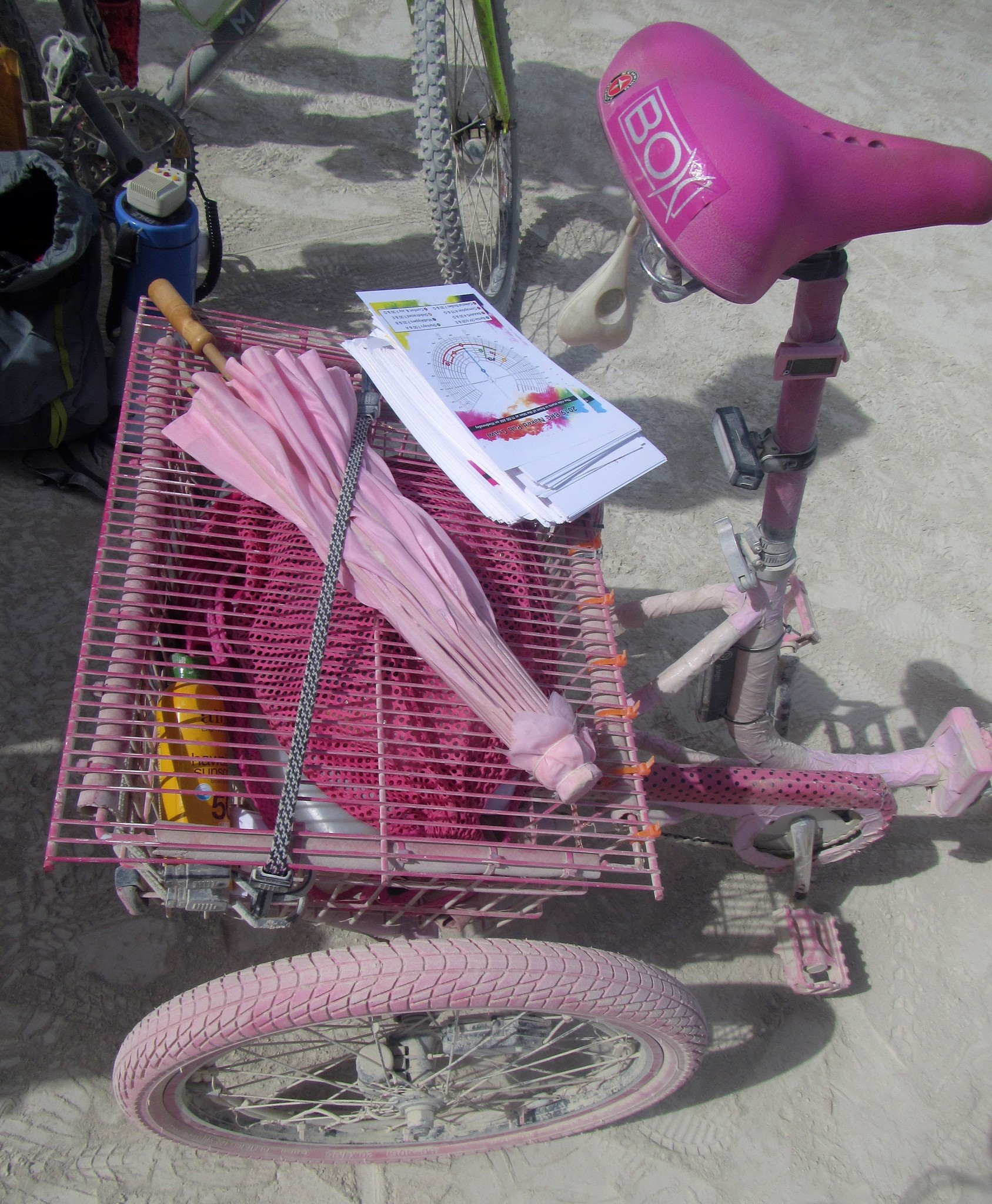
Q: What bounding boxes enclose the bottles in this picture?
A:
[157,652,232,828]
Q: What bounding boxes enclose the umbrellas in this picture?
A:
[148,278,602,807]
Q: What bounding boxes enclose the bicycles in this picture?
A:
[0,1,527,330]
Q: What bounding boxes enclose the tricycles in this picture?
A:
[32,17,991,1166]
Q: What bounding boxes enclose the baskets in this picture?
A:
[94,327,644,935]
